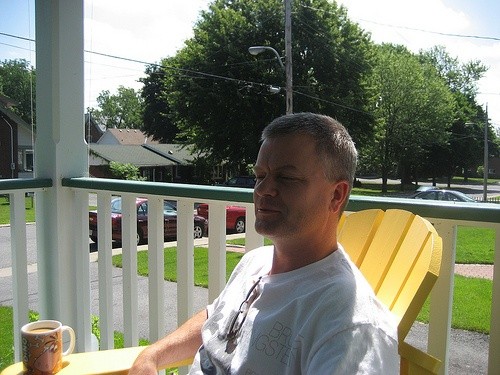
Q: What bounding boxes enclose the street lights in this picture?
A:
[248,45,293,115]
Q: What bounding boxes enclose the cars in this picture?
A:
[197,203,245,232]
[89,198,207,245]
[408,190,479,203]
[415,186,440,193]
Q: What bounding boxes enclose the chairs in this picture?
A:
[0,208,445,375]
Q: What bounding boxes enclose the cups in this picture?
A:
[21,319,76,375]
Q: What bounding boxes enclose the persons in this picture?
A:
[127,111,399,375]
